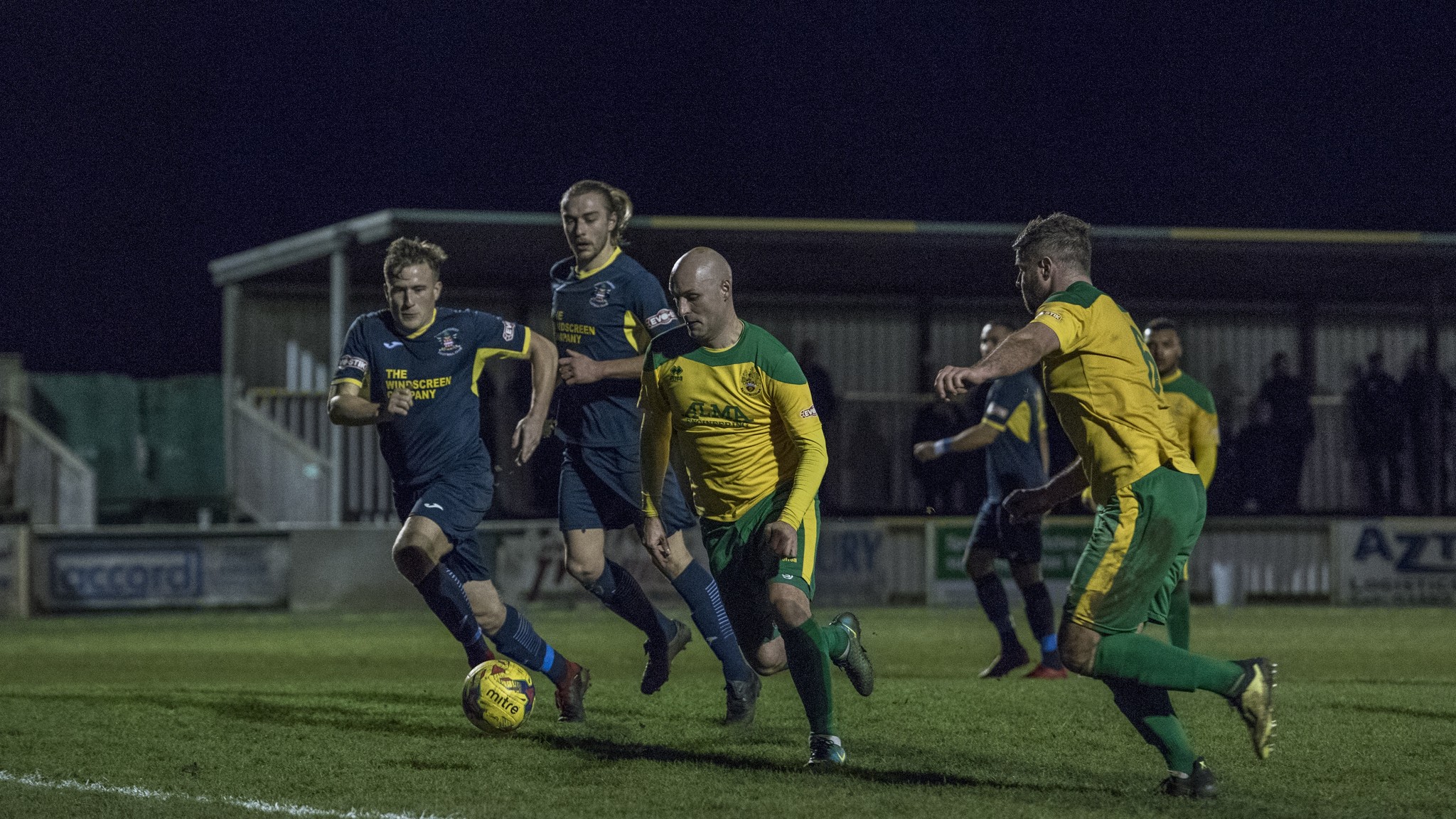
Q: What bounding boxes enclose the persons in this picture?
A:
[637,244,877,766]
[791,337,840,518]
[1136,316,1221,652]
[929,206,1274,797]
[318,232,595,722]
[908,387,977,512]
[1221,337,1455,514]
[907,319,1069,680]
[537,174,765,730]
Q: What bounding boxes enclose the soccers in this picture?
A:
[461,659,536,739]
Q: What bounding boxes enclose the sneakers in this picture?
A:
[831,614,870,697]
[977,647,1030,678]
[638,621,692,693]
[722,665,765,730]
[1163,755,1219,797]
[1025,662,1069,679]
[558,662,592,722]
[804,740,849,770]
[1229,654,1277,759]
